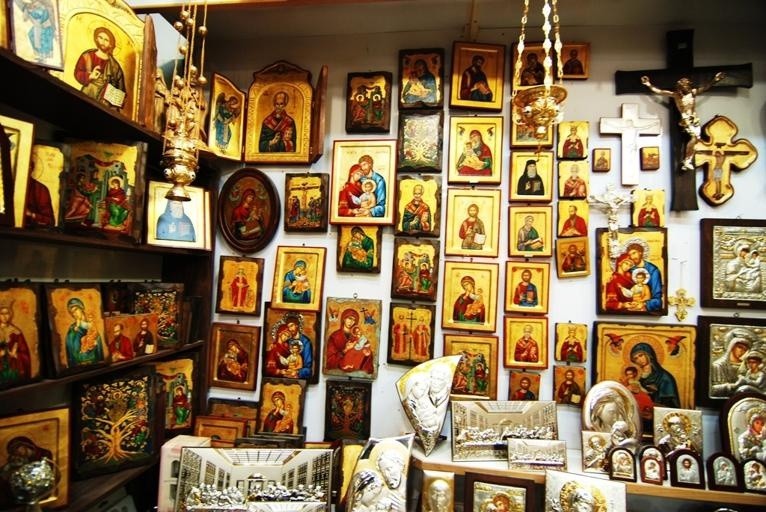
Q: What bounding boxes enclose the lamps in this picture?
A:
[136,13,188,68]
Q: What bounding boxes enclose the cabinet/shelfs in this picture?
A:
[0,46,214,512]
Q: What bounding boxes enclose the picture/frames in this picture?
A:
[127,314,158,357]
[216,168,281,254]
[0,115,33,227]
[130,282,186,350]
[391,236,441,301]
[607,448,637,483]
[450,401,558,462]
[58,137,147,243]
[208,323,262,391]
[640,146,659,170]
[463,471,535,511]
[669,452,704,488]
[631,188,667,226]
[557,121,589,161]
[193,415,248,447]
[21,144,64,228]
[105,316,134,364]
[508,206,553,259]
[553,366,587,408]
[174,446,333,512]
[206,398,261,438]
[6,0,63,72]
[420,469,455,512]
[545,470,626,512]
[591,148,610,172]
[508,370,541,401]
[336,225,383,274]
[700,218,766,311]
[557,41,590,80]
[448,115,502,185]
[397,46,444,113]
[396,355,459,456]
[323,297,382,379]
[329,140,397,222]
[395,175,442,240]
[44,284,111,379]
[1,281,45,390]
[718,392,766,466]
[556,201,589,238]
[341,434,415,512]
[234,438,285,448]
[509,150,554,204]
[345,70,393,134]
[144,180,205,251]
[508,439,567,472]
[582,381,641,457]
[741,459,766,492]
[443,334,497,403]
[554,323,587,364]
[503,315,549,371]
[65,369,159,482]
[249,435,301,449]
[50,0,157,133]
[0,405,72,512]
[149,358,197,438]
[216,256,265,317]
[638,445,668,479]
[259,432,305,448]
[395,111,444,175]
[592,321,699,436]
[323,379,372,446]
[641,457,661,483]
[707,452,744,493]
[443,260,499,334]
[512,42,554,92]
[510,97,554,150]
[696,315,766,410]
[386,302,437,366]
[244,61,327,166]
[450,41,504,111]
[653,408,702,470]
[208,73,245,163]
[555,237,590,278]
[582,431,612,476]
[595,227,667,317]
[505,260,550,315]
[255,377,307,435]
[445,187,501,257]
[558,160,591,200]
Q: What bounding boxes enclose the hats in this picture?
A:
[631,267,650,284]
[627,244,645,252]
[271,392,286,403]
[460,275,475,285]
[289,340,304,355]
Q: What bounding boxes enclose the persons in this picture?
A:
[342,227,377,271]
[402,186,431,232]
[6,433,53,464]
[519,216,543,251]
[156,200,194,240]
[489,495,510,512]
[513,325,539,362]
[511,377,534,400]
[76,25,128,107]
[347,80,383,124]
[618,268,651,312]
[721,235,763,290]
[606,255,635,312]
[640,71,725,171]
[402,59,438,104]
[521,52,545,87]
[287,182,325,230]
[227,267,253,310]
[282,254,312,303]
[713,329,766,398]
[709,150,726,200]
[563,244,584,272]
[396,249,432,296]
[0,296,31,381]
[217,338,251,382]
[646,462,657,478]
[626,243,664,315]
[656,415,699,453]
[23,0,53,62]
[350,179,377,218]
[461,54,494,103]
[338,165,361,217]
[564,327,583,360]
[107,323,134,361]
[564,126,584,156]
[63,297,104,368]
[452,276,488,323]
[738,410,766,458]
[457,126,495,180]
[394,313,429,357]
[562,205,586,236]
[749,464,765,488]
[104,178,130,226]
[458,204,486,250]
[515,270,538,307]
[518,160,545,195]
[172,384,191,428]
[559,370,579,400]
[583,435,610,464]
[71,308,100,353]
[24,165,53,229]
[261,90,302,155]
[564,165,586,197]
[638,196,659,226]
[597,150,608,168]
[260,388,293,432]
[619,366,656,415]
[453,350,489,393]
[645,152,658,168]
[717,461,730,484]
[230,182,265,240]
[358,155,387,217]
[134,319,155,358]
[265,311,314,376]
[630,342,680,410]
[213,89,238,155]
[561,47,581,76]
[67,174,90,225]
[678,459,696,481]
[327,305,376,375]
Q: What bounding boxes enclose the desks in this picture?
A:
[409,441,766,512]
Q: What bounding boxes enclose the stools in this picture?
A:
[158,435,211,512]
